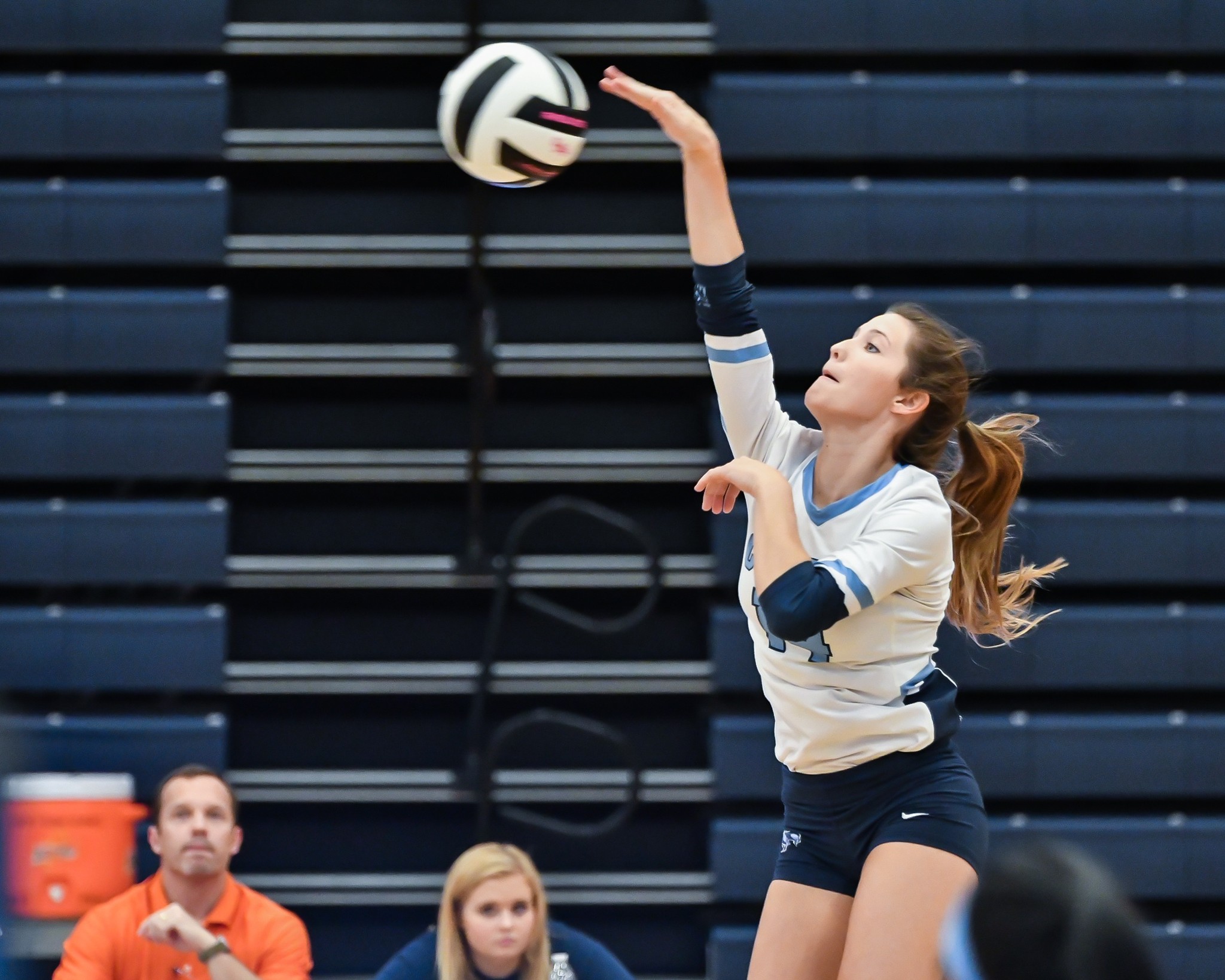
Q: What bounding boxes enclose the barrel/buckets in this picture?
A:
[0,773,146,917]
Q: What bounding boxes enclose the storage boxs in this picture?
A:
[1,772,149,919]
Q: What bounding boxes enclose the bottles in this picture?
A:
[548,952,577,980]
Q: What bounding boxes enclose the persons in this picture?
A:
[600,63,1071,980]
[51,766,313,980]
[366,842,639,980]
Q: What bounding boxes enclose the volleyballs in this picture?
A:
[437,42,590,188]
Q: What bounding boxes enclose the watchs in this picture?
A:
[197,936,231,964]
